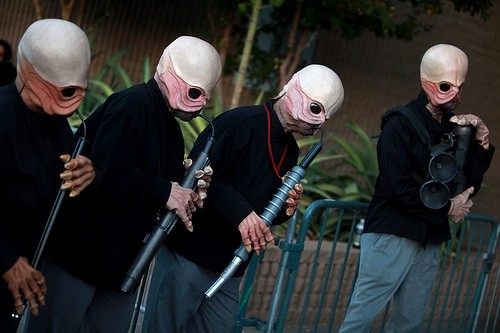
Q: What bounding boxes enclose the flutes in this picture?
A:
[119,115,216,293]
[205,127,324,299]
[5,109,86,333]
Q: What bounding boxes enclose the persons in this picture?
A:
[129,64,345,333]
[24,35,223,333]
[0,39,17,86]
[337,44,496,333]
[0,18,96,333]
